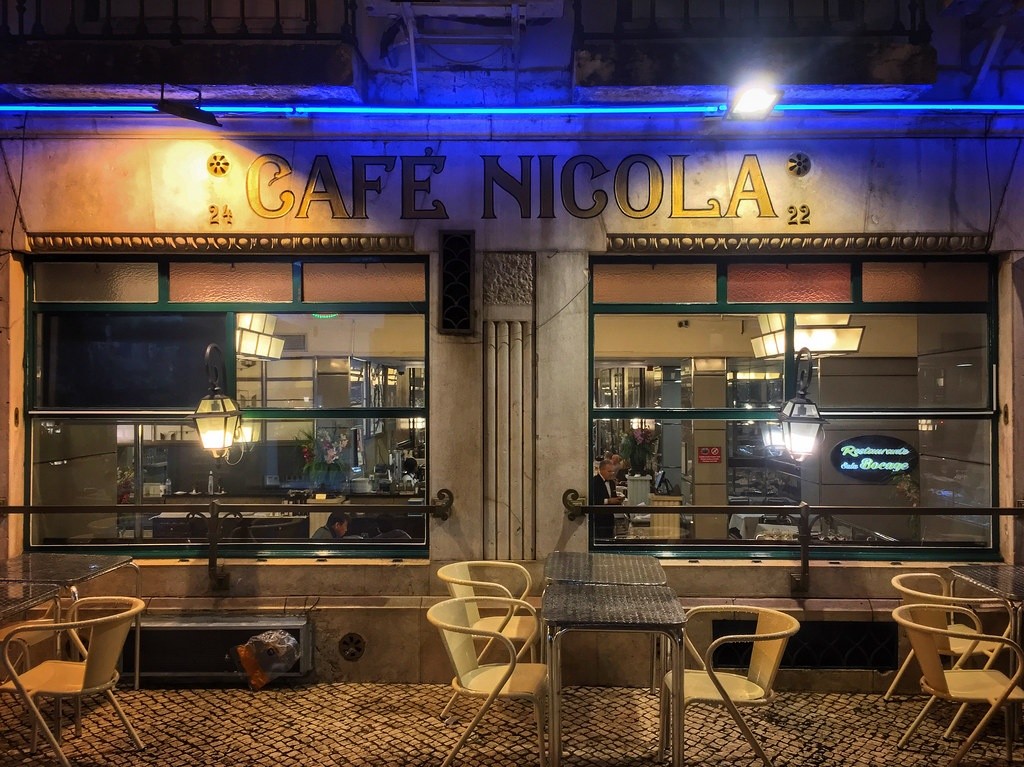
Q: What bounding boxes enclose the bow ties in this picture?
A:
[605,480,610,482]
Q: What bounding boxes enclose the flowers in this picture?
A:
[620,428,658,460]
[294,422,355,481]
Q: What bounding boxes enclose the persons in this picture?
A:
[612,454,628,481]
[310,511,349,539]
[593,460,624,538]
[401,458,420,488]
[604,451,614,460]
[374,514,412,538]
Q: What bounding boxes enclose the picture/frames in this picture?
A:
[316,426,358,467]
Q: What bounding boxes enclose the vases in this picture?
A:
[312,476,337,498]
[628,456,646,477]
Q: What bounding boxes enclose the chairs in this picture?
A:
[891,603,1024,767]
[762,497,791,507]
[437,561,547,725]
[727,496,749,506]
[657,604,801,767]
[758,515,793,526]
[0,596,146,767]
[426,596,550,767]
[883,573,1012,740]
[0,619,66,726]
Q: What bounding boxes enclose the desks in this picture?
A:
[0,580,63,747]
[540,584,688,767]
[152,512,310,539]
[948,565,1024,741]
[624,493,991,540]
[0,552,141,738]
[328,495,427,525]
[542,551,671,749]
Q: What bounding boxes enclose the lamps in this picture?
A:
[235,313,285,361]
[191,342,244,461]
[237,392,263,454]
[152,77,223,128]
[722,71,785,121]
[350,367,398,387]
[750,314,865,361]
[779,347,831,462]
[759,363,786,456]
